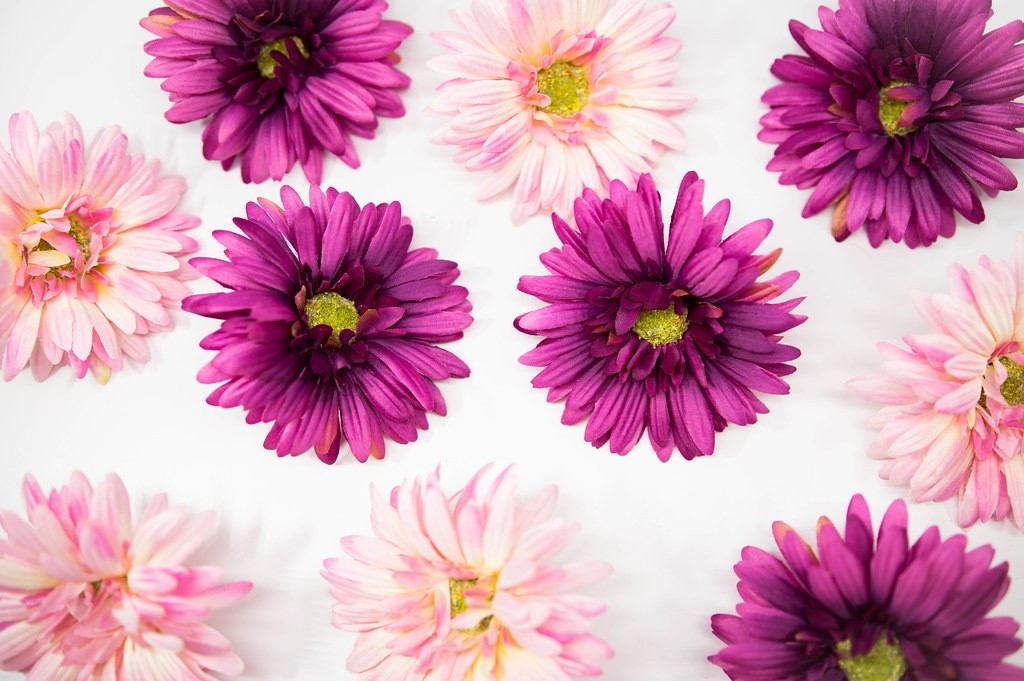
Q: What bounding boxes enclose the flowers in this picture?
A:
[427,1,696,228]
[317,457,617,681]
[0,467,258,681]
[179,183,477,468]
[0,109,206,392]
[753,1,1023,253]
[839,236,1024,537]
[704,492,1024,681]
[138,0,415,185]
[511,172,808,465]
